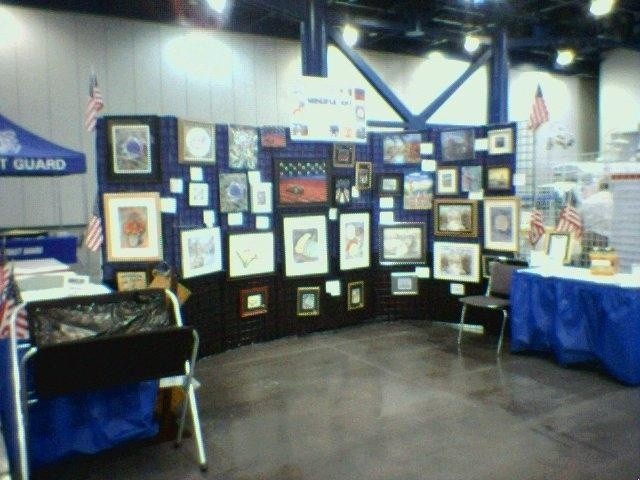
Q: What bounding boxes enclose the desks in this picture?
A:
[14,285,207,480]
[507,264,640,385]
[0,231,174,471]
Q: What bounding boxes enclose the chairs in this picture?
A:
[458,258,529,356]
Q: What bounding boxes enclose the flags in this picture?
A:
[558,190,583,237]
[83,70,105,131]
[527,203,544,244]
[0,238,32,339]
[527,84,550,130]
[83,197,105,252]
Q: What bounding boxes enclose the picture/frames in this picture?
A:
[543,228,574,262]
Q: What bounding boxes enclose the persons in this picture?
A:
[580,177,611,248]
